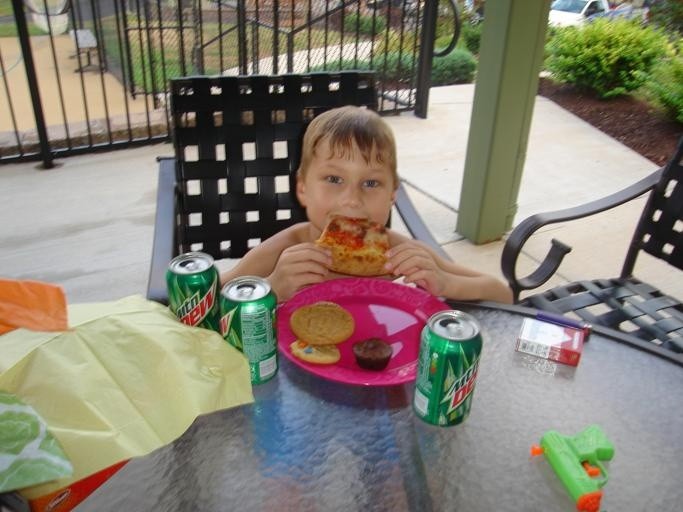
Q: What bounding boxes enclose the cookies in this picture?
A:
[290,342,340,365]
[289,302,354,344]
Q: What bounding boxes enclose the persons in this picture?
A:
[215,106,516,306]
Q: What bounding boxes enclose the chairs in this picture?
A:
[145,72,457,306]
[501,136,683,354]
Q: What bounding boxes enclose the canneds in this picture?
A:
[413,308,482,426]
[165,253,220,334]
[220,275,279,387]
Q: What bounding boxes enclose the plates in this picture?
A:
[277,278,454,385]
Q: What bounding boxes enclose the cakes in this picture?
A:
[351,338,393,370]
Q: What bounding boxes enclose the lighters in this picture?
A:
[535,310,592,338]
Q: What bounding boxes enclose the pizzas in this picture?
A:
[315,216,390,277]
